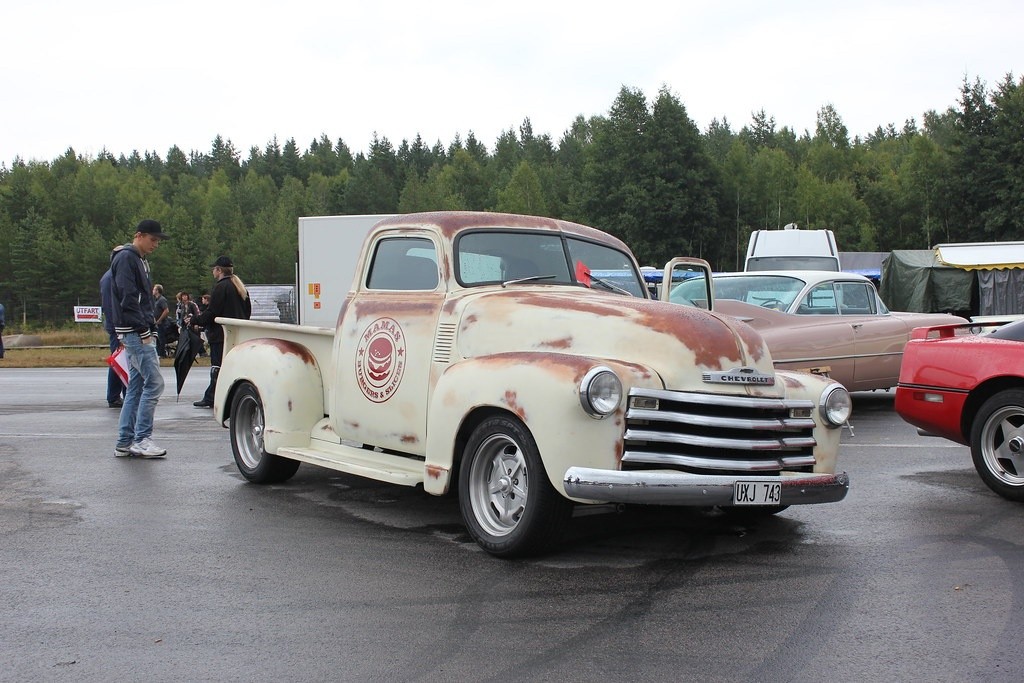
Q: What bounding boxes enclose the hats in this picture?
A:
[110,245,125,262]
[137,220,170,241]
[208,255,233,267]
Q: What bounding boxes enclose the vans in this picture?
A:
[745,225,842,318]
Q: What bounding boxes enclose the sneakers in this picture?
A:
[115,446,139,457]
[129,436,167,456]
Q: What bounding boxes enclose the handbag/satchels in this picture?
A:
[163,315,179,344]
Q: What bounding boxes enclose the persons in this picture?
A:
[99,220,172,457]
[184,256,251,408]
[152,284,211,359]
[0,303,5,359]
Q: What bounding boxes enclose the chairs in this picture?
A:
[505,259,540,281]
[392,256,437,290]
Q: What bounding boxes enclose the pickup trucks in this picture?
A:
[215,209,851,557]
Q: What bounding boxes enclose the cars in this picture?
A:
[893,313,1023,503]
[575,265,717,304]
[657,270,973,402]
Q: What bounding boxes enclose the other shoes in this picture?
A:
[193,398,214,408]
[158,354,170,359]
[108,397,124,408]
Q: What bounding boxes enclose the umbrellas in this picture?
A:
[107,344,129,389]
[174,316,204,402]
